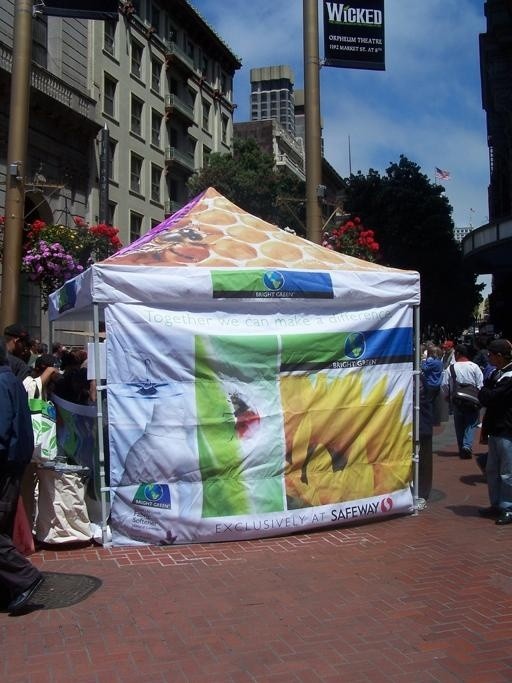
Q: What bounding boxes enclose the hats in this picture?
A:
[442,340,453,348]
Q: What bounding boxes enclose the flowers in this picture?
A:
[20,213,125,311]
[323,211,382,265]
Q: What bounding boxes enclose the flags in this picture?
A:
[435,166,451,179]
[322,0,387,71]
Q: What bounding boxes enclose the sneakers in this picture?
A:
[8,572,45,613]
[418,498,427,510]
[496,513,511,526]
[479,507,496,516]
[462,448,472,459]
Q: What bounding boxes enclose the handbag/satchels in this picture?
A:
[450,364,482,420]
[29,378,57,463]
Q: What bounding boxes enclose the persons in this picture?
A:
[474,337,511,528]
[0,335,47,614]
[0,314,111,487]
[413,319,498,497]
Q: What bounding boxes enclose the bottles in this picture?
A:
[110,403,203,543]
